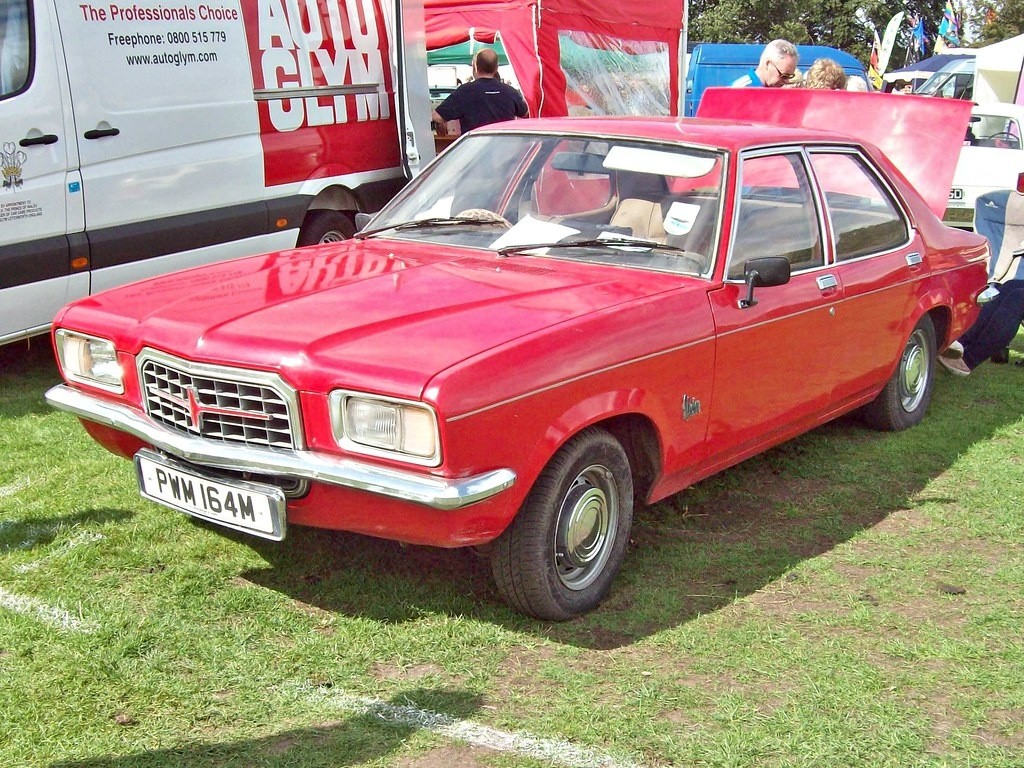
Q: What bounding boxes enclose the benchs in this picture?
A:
[613,195,810,269]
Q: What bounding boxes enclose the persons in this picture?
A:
[432,48,530,138]
[934,279,1024,376]
[726,39,856,206]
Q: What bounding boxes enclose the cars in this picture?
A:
[944,103,1024,230]
[883,48,975,102]
[46,83,1001,624]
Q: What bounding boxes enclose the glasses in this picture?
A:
[771,61,795,79]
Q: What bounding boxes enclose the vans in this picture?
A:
[683,43,877,120]
[0,0,418,348]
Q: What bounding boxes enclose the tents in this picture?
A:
[972,32,1024,139]
[401,0,691,158]
[881,46,979,102]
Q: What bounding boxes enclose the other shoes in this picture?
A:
[941,340,964,358]
[936,354,971,377]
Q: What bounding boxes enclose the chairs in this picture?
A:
[974,189,1024,368]
